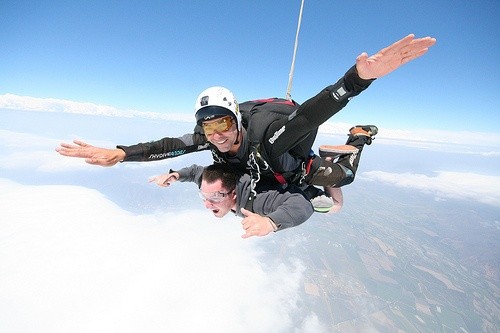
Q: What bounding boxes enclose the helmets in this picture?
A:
[194,87,242,131]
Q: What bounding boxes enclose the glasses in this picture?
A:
[202,117,234,135]
[199,188,235,202]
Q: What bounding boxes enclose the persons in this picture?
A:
[55,34,439,188]
[147,145,360,239]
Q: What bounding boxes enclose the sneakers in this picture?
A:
[319,145,359,163]
[349,125,378,145]
